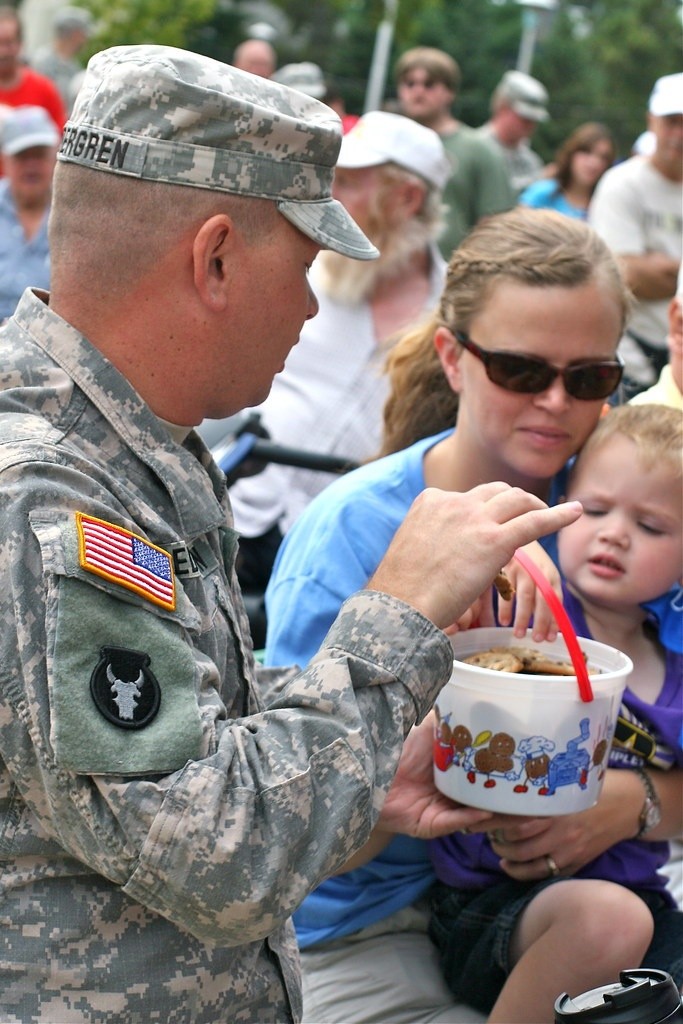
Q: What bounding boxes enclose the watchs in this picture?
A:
[632,767,663,840]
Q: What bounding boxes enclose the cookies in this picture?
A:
[456,645,604,676]
[493,567,515,602]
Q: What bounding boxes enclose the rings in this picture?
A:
[545,856,559,876]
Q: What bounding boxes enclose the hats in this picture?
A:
[650,74,683,117]
[57,44,381,260]
[0,106,58,155]
[633,132,657,156]
[334,111,453,190]
[497,70,551,123]
[268,61,326,98]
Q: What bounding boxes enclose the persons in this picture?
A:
[394,46,515,262]
[426,403,683,1024]
[232,38,276,78]
[585,72,683,406]
[0,5,66,136]
[0,103,61,322]
[30,6,95,95]
[515,122,616,219]
[228,111,453,595]
[479,70,557,190]
[262,207,683,1024]
[0,44,584,1024]
[628,258,683,412]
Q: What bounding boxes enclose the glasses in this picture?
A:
[456,333,624,401]
[403,77,445,90]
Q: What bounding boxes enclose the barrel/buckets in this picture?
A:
[432,548,633,817]
